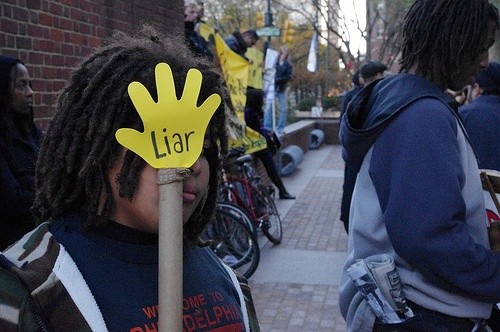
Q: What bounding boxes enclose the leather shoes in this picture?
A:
[279,191,295,199]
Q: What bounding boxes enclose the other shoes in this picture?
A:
[278,128,284,134]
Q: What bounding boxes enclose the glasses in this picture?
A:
[251,37,255,45]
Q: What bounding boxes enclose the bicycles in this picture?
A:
[193,147,283,279]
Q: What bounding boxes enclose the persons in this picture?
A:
[338,0,500,332]
[223,28,260,64]
[245,86,296,200]
[0,56,45,253]
[0,23,260,332]
[264,44,292,135]
